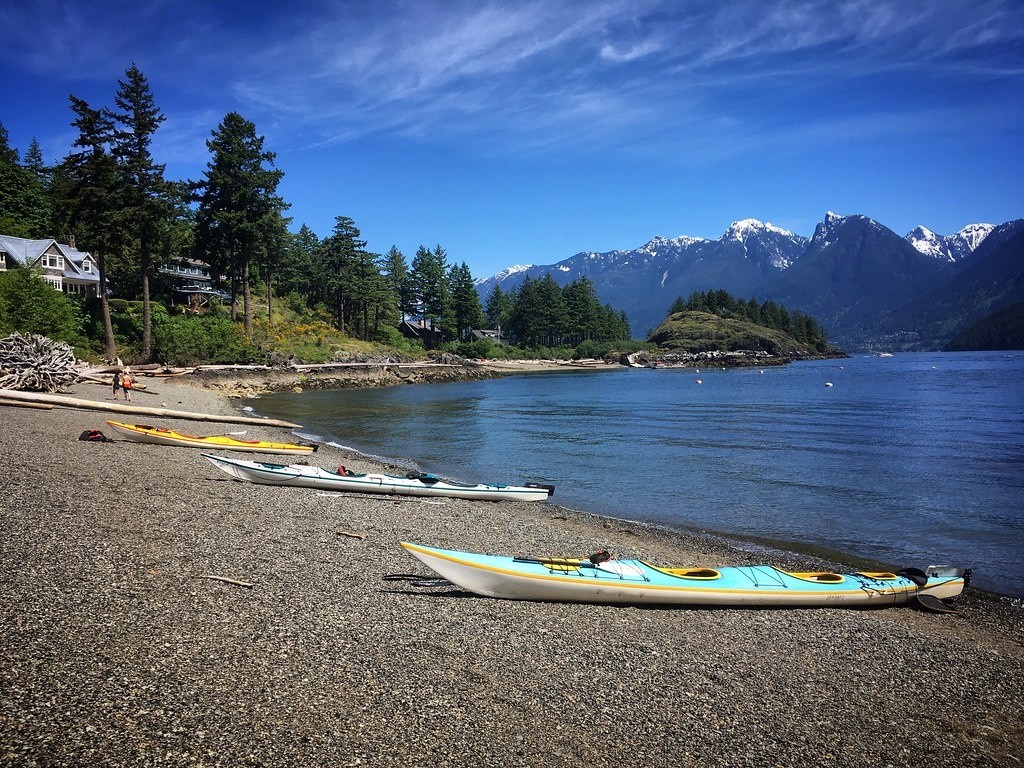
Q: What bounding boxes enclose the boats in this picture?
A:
[200,451,556,502]
[106,419,320,456]
[395,540,975,618]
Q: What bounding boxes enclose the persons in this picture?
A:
[113,374,119,400]
[123,371,131,401]
